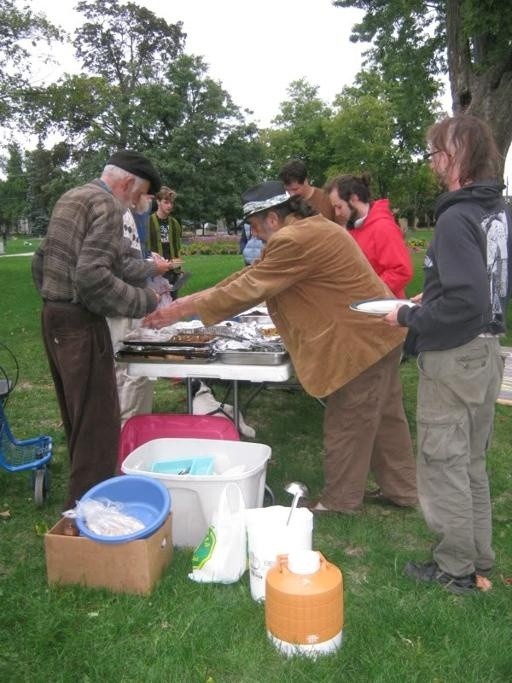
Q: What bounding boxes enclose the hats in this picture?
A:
[104,148,162,196]
[234,179,304,232]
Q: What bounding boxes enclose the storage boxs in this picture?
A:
[43,503,174,596]
[127,436,272,548]
[120,412,237,478]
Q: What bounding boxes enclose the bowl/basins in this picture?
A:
[73,476,171,542]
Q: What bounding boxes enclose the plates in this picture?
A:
[349,298,419,318]
[150,258,185,268]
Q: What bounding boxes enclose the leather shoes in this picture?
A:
[287,497,343,516]
[362,488,412,509]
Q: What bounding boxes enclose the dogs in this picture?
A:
[185,377,257,440]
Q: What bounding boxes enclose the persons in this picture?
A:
[325,174,413,298]
[31,149,160,517]
[368,115,512,589]
[141,180,419,513]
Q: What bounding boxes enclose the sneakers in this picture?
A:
[402,558,493,596]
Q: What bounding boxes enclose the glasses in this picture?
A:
[423,149,453,168]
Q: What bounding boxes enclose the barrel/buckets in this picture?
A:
[265,551,343,661]
[244,505,313,603]
